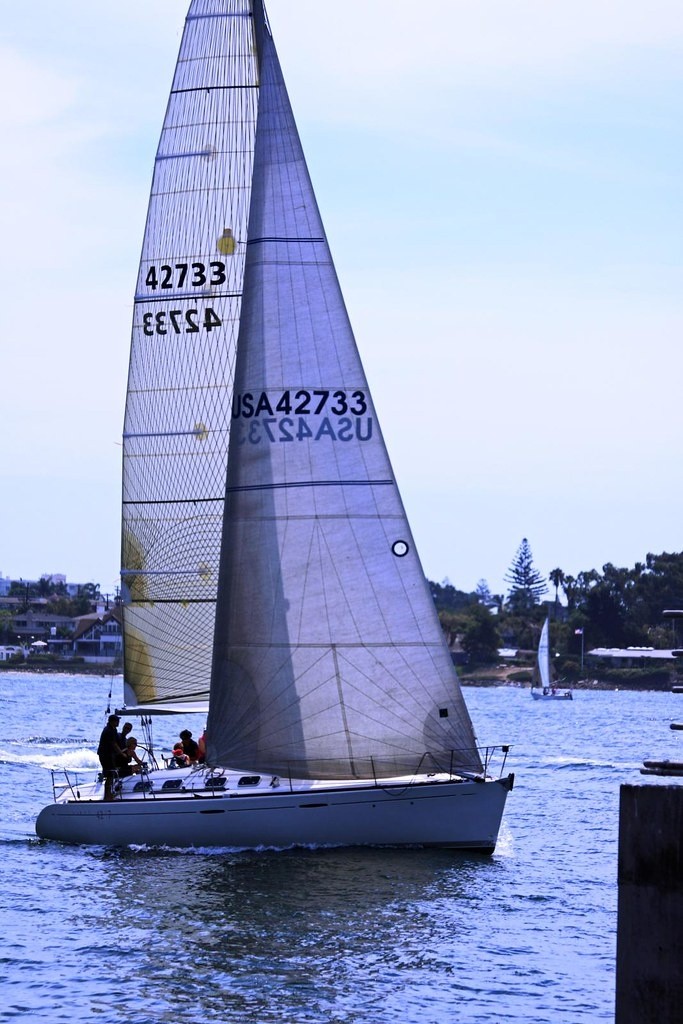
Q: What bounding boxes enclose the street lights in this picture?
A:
[17,634,35,649]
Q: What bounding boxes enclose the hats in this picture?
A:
[172,749,183,756]
[108,715,121,721]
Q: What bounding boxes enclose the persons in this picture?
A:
[552,684,556,695]
[97,714,149,800]
[543,686,549,695]
[173,724,206,768]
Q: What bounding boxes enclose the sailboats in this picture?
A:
[530,617,573,700]
[35,0,515,856]
